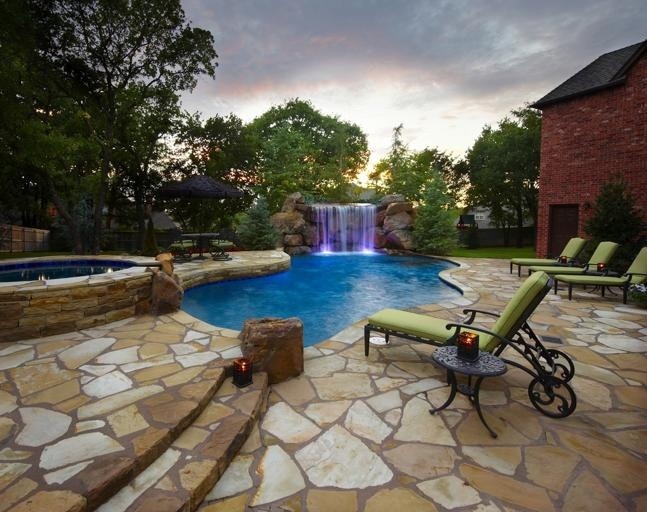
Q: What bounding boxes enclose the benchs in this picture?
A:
[459,215,479,231]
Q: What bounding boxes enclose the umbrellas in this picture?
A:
[157,174,244,255]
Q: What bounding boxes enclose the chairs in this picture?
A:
[206,239,234,261]
[363,271,576,419]
[170,226,191,268]
[510,238,647,305]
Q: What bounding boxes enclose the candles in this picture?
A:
[231,355,252,377]
[457,327,482,347]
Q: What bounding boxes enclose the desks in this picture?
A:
[180,230,219,260]
[430,346,508,442]
